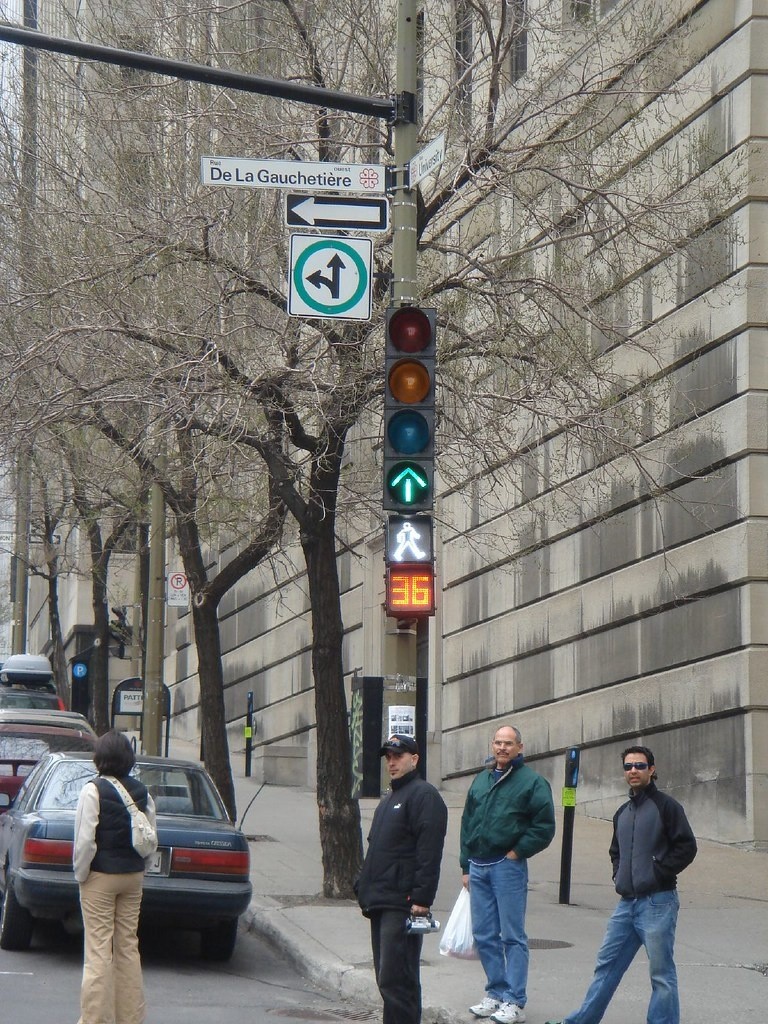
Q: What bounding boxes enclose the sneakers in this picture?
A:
[468,997,506,1016]
[489,1002,526,1024]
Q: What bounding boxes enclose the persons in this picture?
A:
[353,734,427,1024]
[458,725,555,1023]
[73,729,157,1024]
[558,746,698,1024]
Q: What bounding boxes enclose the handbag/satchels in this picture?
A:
[131,809,158,858]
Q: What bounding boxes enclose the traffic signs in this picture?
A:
[202,155,387,193]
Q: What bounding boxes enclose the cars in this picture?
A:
[0,654,66,714]
[0,750,255,971]
[1,707,107,816]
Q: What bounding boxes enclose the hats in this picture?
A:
[380,735,420,756]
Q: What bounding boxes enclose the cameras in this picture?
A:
[405,912,441,935]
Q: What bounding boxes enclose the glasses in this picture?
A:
[492,740,518,747]
[624,763,651,771]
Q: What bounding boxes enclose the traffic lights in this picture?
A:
[384,514,435,568]
[384,460,433,513]
[386,307,440,460]
[385,568,437,619]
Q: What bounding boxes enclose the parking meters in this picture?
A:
[557,745,585,904]
[243,690,253,776]
